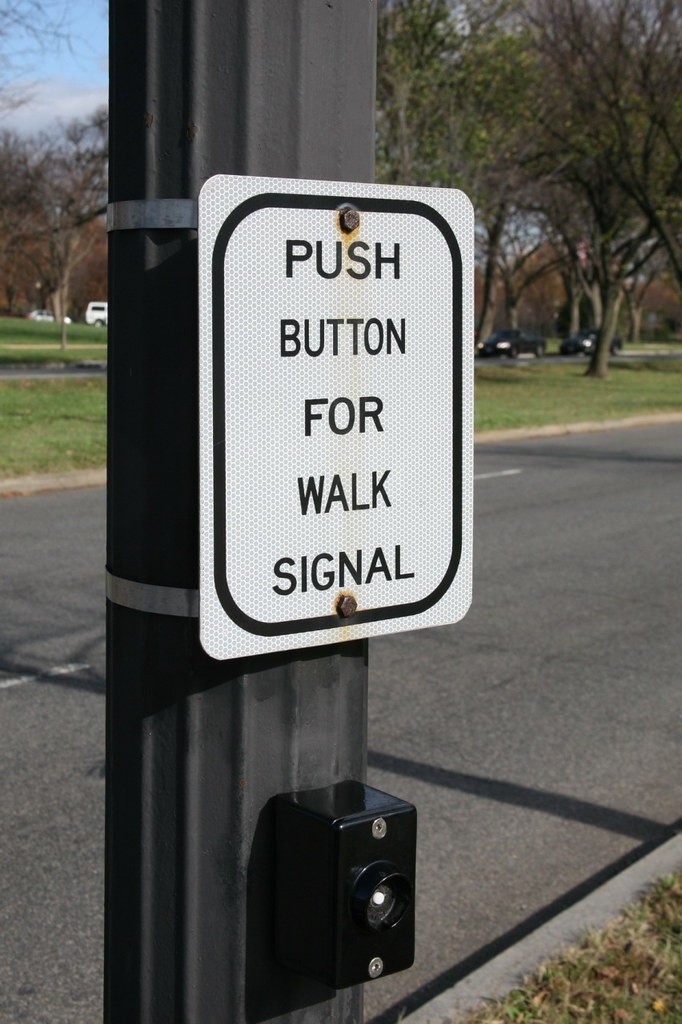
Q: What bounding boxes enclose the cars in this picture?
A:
[558,327,625,355]
[478,329,548,359]
[25,309,72,324]
[85,302,108,327]
